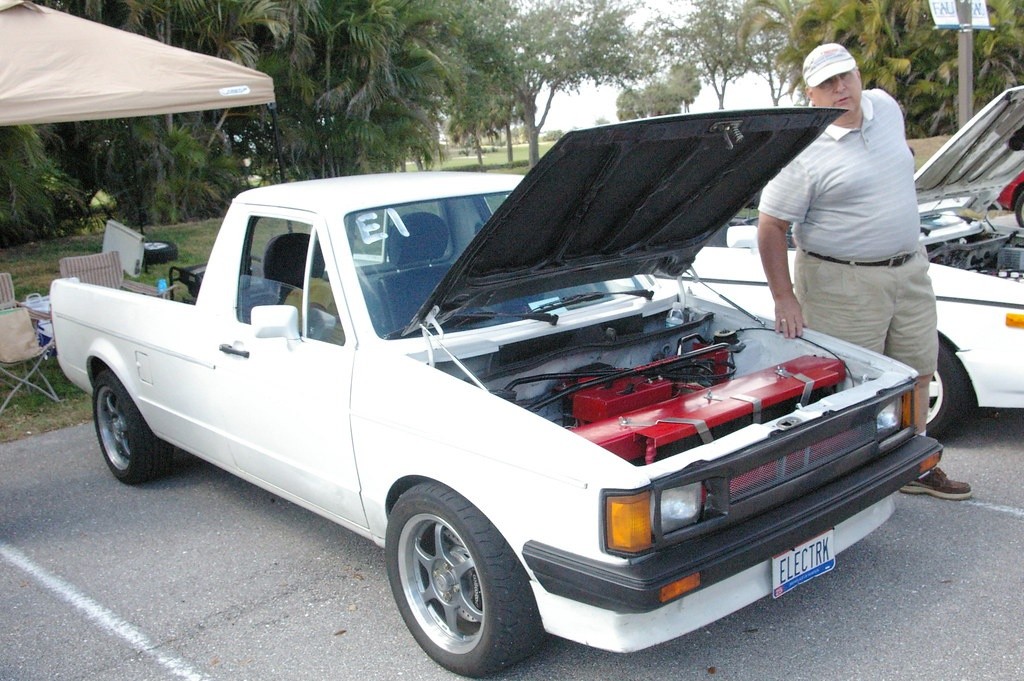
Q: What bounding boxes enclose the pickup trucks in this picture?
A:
[674,85,1024,446]
[47,105,943,681]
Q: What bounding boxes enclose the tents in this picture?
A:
[1,0,296,275]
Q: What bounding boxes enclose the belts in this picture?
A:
[802,249,917,266]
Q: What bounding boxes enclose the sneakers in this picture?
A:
[900,468,971,501]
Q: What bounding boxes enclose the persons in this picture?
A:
[757,42,973,500]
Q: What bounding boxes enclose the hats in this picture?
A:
[802,43,856,87]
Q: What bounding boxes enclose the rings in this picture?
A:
[781,319,786,322]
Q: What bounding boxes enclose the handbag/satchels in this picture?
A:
[0,296,43,363]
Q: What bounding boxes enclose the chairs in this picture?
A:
[247,232,345,347]
[0,272,60,414]
[59,250,171,301]
[378,213,453,336]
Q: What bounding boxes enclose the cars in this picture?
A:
[997,170,1024,229]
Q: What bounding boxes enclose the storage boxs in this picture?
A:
[21,292,57,356]
